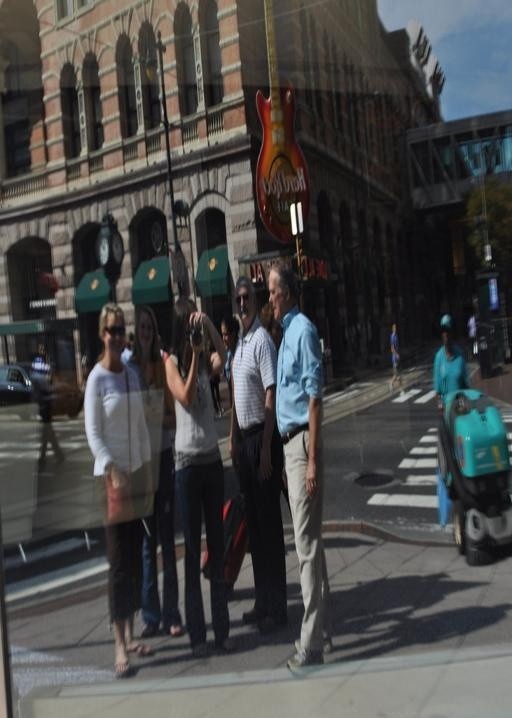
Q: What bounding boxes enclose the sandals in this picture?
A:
[114,642,153,677]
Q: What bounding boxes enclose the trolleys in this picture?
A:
[434,383,512,570]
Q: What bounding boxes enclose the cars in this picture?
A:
[0,360,84,423]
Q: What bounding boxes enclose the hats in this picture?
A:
[441,314,452,327]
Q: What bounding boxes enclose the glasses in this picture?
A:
[105,326,125,335]
[234,293,252,302]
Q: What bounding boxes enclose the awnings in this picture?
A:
[196,243,230,297]
[131,248,169,304]
[75,259,112,311]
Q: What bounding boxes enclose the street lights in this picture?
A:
[286,190,307,315]
[143,29,187,303]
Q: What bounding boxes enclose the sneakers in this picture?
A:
[285,631,332,677]
[242,610,289,632]
[140,623,182,637]
[190,635,237,655]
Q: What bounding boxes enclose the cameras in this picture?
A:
[190,327,202,346]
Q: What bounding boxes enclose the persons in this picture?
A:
[128,308,184,637]
[467,314,480,360]
[389,323,405,396]
[261,301,283,343]
[30,375,64,468]
[85,303,156,674]
[269,267,328,676]
[166,297,237,658]
[202,318,239,417]
[229,277,287,629]
[432,313,468,527]
